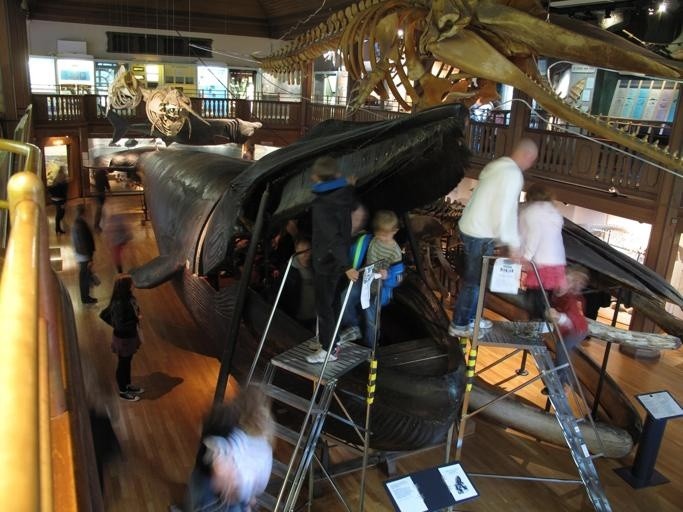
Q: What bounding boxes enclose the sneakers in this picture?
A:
[119,384,145,402]
[304,342,340,363]
[447,320,484,339]
[541,321,554,334]
[469,316,494,329]
[562,383,571,399]
[541,386,550,396]
[339,327,361,346]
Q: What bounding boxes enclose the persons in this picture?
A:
[299,154,360,363]
[49,167,67,234]
[89,172,110,230]
[335,208,402,349]
[515,184,568,322]
[106,272,145,402]
[202,382,277,512]
[69,204,97,304]
[446,137,536,338]
[539,269,590,396]
[183,405,268,511]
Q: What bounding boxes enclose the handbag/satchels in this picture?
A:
[99,304,114,328]
